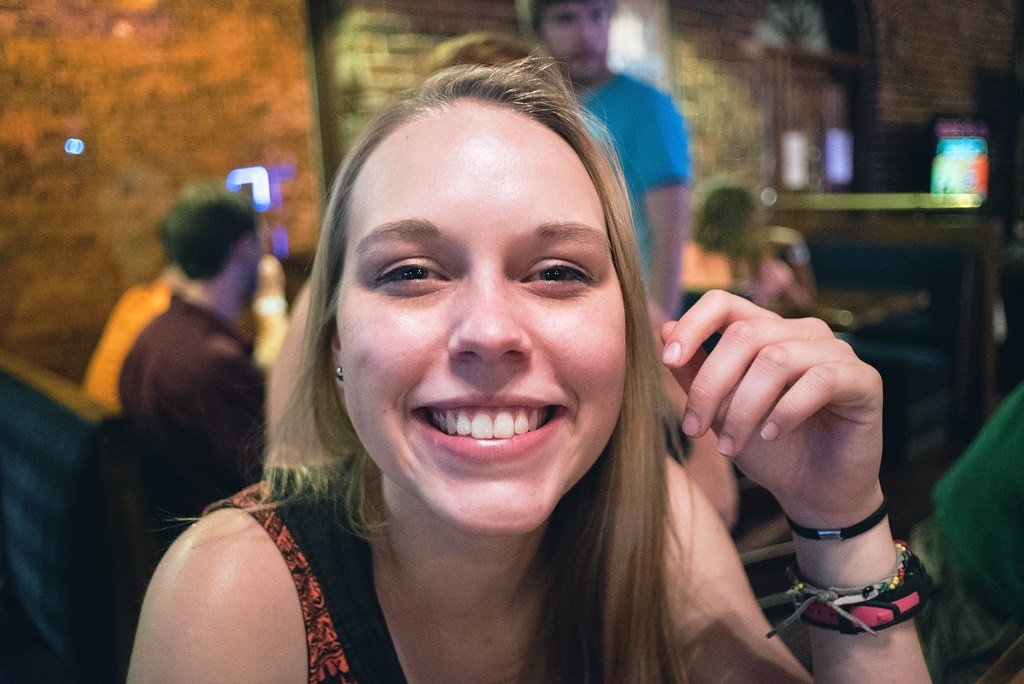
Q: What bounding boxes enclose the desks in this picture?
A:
[813,290,931,329]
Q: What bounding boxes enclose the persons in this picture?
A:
[533,0,694,314]
[680,183,819,316]
[126,65,935,684]
[914,382,1024,684]
[87,182,290,552]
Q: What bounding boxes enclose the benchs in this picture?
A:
[0,351,129,675]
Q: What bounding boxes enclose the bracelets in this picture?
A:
[783,486,888,540]
[766,538,932,638]
